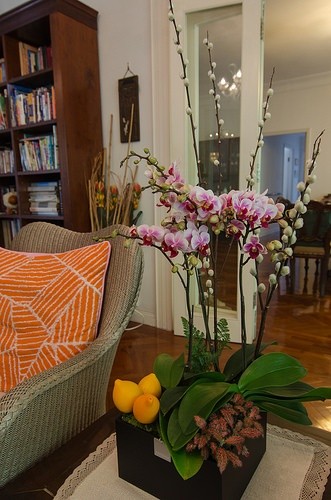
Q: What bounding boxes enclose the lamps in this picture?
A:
[217,62,242,101]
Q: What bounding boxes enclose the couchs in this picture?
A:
[0,221,145,490]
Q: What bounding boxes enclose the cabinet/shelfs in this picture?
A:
[0,1,103,249]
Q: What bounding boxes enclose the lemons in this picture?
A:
[133,393,160,424]
[137,372,161,399]
[112,379,144,414]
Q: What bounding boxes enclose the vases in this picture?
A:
[114,407,267,499]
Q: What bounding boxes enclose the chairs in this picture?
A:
[285,199,329,288]
[320,193,331,206]
[274,196,293,246]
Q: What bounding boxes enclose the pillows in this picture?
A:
[1,241,112,398]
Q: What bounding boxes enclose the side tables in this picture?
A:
[43,410,331,499]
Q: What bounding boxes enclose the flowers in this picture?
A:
[92,1,324,479]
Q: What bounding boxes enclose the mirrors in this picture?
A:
[185,1,244,315]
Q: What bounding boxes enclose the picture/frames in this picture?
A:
[118,75,141,143]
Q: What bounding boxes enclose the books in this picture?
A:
[0,147,14,175]
[26,180,63,217]
[0,57,6,83]
[0,218,21,250]
[5,83,56,127]
[0,186,16,208]
[18,41,52,77]
[17,125,60,173]
[0,89,8,130]
